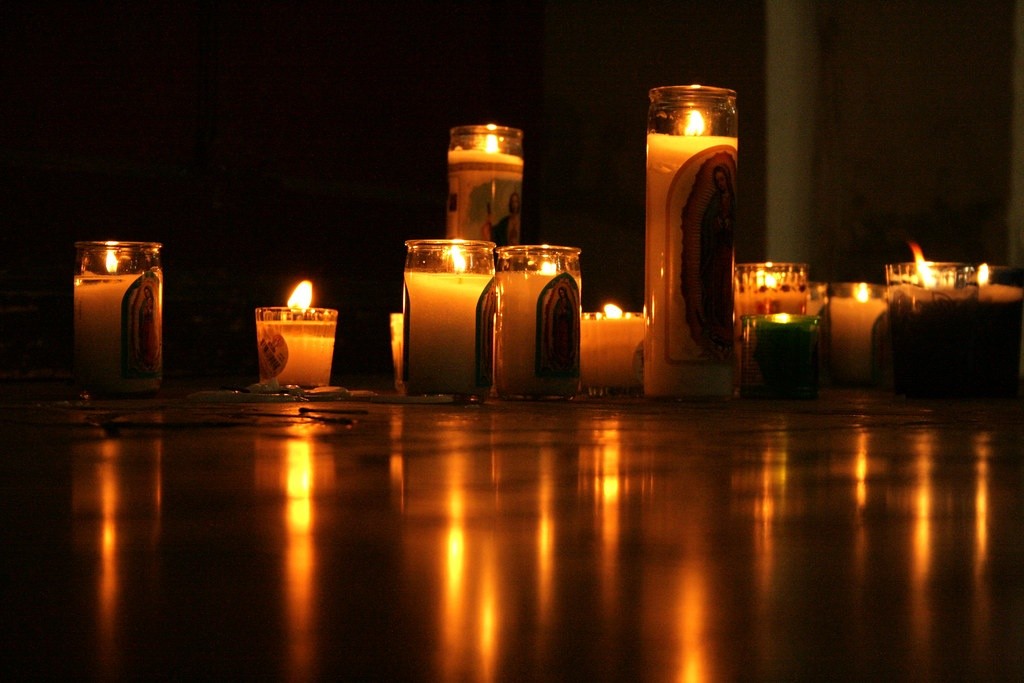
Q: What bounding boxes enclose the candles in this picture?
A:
[75,272,162,400]
[403,152,582,404]
[583,135,1023,401]
[255,308,338,389]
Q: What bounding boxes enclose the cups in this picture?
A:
[404,240,497,402]
[389,312,404,393]
[255,308,338,388]
[582,86,1024,394]
[447,126,522,244]
[72,242,164,392]
[496,244,581,399]
